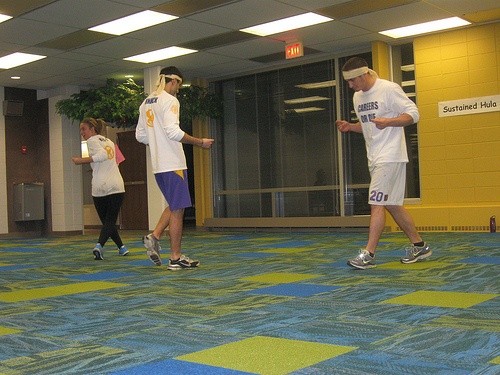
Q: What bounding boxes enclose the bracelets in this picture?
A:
[199,138,203,148]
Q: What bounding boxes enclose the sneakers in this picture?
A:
[116,245,130,256]
[142,232,162,266]
[347,247,376,269]
[400,242,432,264]
[167,254,200,270]
[93,243,103,260]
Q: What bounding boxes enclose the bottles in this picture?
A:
[490,216,496,233]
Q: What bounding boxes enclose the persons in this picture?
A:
[135,65,215,271]
[71,116,130,260]
[335,57,432,269]
